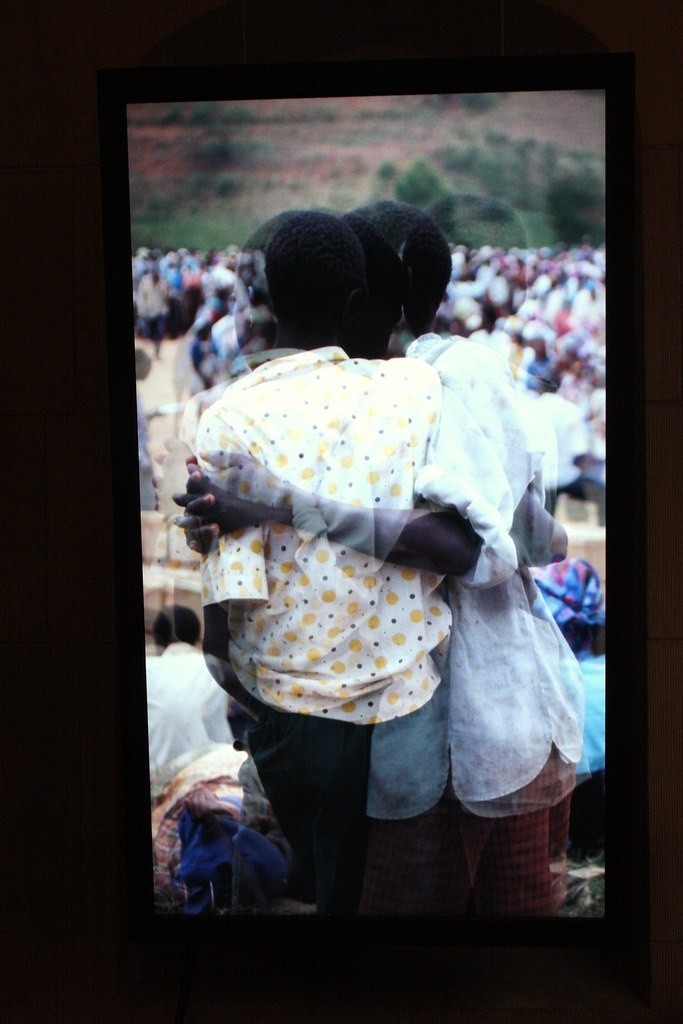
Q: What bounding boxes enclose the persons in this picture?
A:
[135,201,606,920]
